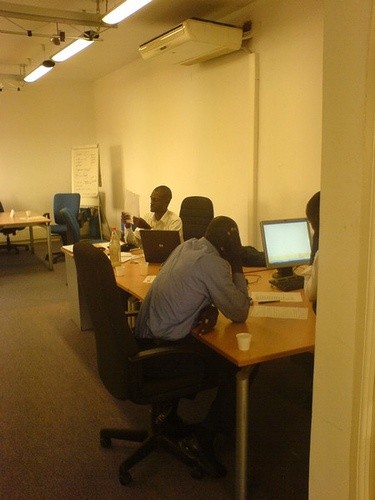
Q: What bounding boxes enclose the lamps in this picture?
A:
[50,30,99,63]
[100,0,152,27]
[23,44,55,83]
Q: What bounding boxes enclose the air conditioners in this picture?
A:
[139,15,245,67]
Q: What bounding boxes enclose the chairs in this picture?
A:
[0,201,28,255]
[43,193,80,263]
[74,240,228,487]
[179,196,214,242]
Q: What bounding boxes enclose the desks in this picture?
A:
[60,240,316,500]
[0,210,51,255]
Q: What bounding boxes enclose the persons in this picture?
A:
[134,217,261,478]
[119,186,184,249]
[303,191,319,312]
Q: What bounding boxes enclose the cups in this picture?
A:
[115,265,126,277]
[139,262,148,275]
[26,211,31,216]
[11,209,15,217]
[235,333,252,351]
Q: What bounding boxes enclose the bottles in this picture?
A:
[125,223,135,246]
[109,226,121,267]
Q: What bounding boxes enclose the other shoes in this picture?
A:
[181,436,227,479]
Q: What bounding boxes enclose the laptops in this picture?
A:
[139,229,180,263]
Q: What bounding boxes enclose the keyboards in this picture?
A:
[269,276,304,291]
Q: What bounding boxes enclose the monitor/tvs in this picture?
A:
[260,217,315,277]
